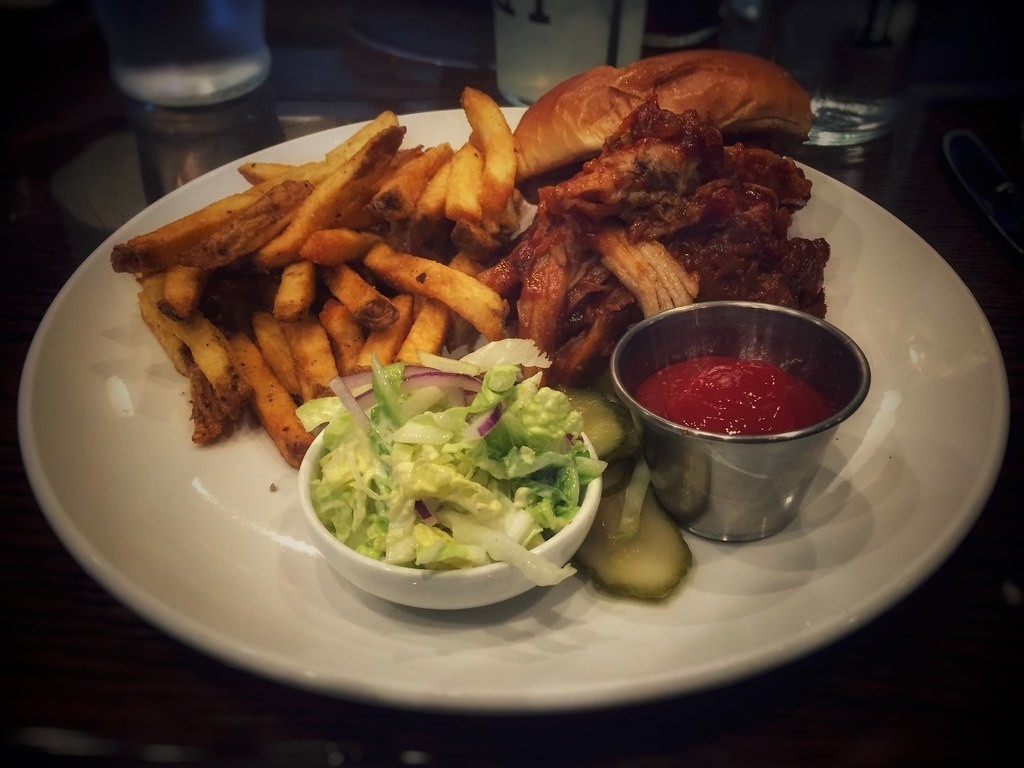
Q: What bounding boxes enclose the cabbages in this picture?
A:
[293,338,650,586]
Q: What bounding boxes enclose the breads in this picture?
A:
[507,48,812,201]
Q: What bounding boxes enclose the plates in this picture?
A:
[345,10,493,71]
[17,109,1009,716]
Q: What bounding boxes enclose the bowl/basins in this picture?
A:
[298,431,604,608]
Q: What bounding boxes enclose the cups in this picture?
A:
[612,301,872,540]
[760,0,922,147]
[97,0,271,109]
[644,0,726,51]
[495,0,649,106]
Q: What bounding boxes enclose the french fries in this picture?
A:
[111,88,520,467]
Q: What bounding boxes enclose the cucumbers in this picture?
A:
[555,382,696,601]
